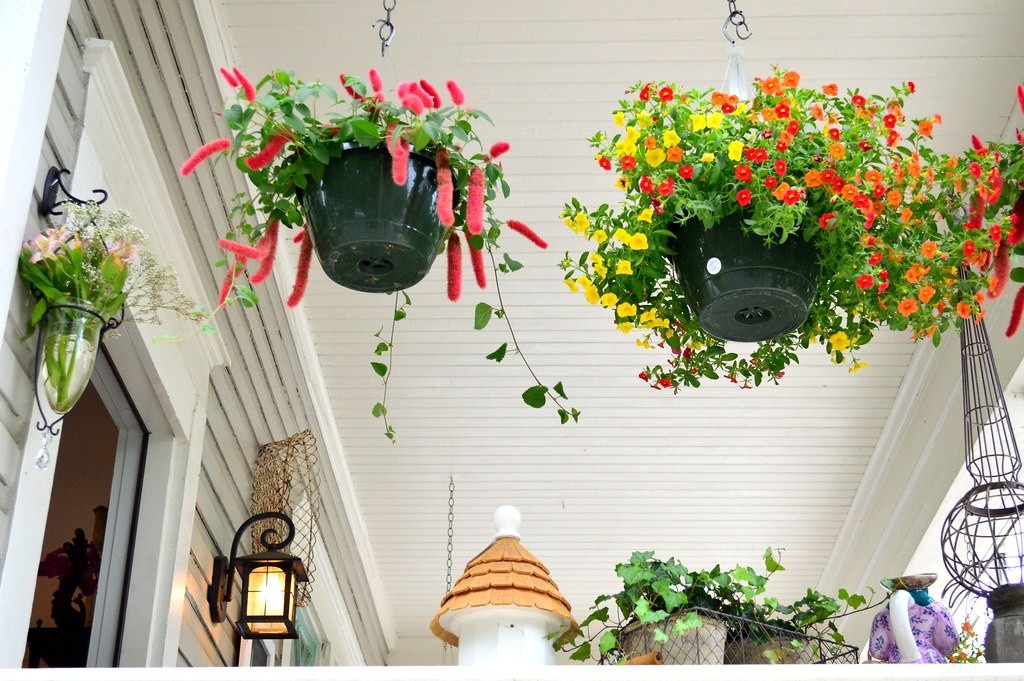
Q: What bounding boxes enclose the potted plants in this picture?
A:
[543,545,907,665]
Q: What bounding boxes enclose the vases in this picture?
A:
[297,141,456,293]
[664,215,820,342]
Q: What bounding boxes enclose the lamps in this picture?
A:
[206,512,311,640]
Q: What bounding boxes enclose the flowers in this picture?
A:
[21,203,208,409]
[177,63,583,447]
[558,64,1020,394]
